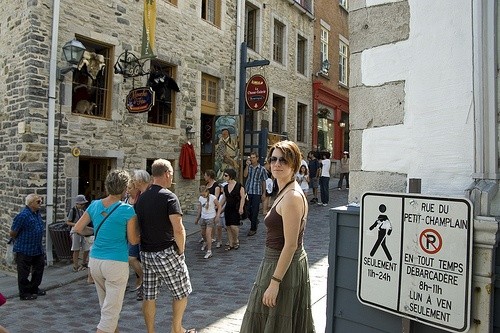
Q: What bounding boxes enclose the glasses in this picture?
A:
[36,200,40,204]
[343,153,348,155]
[225,175,229,177]
[269,155,286,164]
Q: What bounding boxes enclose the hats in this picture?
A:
[74,194,89,204]
[220,182,228,186]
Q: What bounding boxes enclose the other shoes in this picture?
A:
[135,277,141,290]
[20,295,38,300]
[136,292,143,301]
[310,198,318,203]
[228,244,234,250]
[32,288,46,296]
[317,202,328,206]
[216,241,223,247]
[211,238,216,243]
[204,250,213,259]
[234,240,241,250]
[248,230,257,236]
[201,242,207,250]
[257,219,260,226]
[198,237,203,242]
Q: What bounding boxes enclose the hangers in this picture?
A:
[181,141,196,151]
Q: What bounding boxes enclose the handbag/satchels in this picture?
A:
[5,236,17,268]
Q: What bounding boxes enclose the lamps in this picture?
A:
[150,70,168,84]
[61,37,84,74]
[322,109,346,127]
[186,126,195,140]
[319,60,331,74]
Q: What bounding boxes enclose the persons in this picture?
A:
[237,141,316,333]
[0,292,9,333]
[72,166,142,333]
[6,193,46,300]
[193,148,350,258]
[128,159,197,333]
[64,195,92,272]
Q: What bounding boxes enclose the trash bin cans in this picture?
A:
[48,221,79,264]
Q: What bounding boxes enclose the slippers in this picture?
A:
[72,264,81,272]
[185,328,197,333]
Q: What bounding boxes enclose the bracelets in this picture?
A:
[271,275,282,283]
